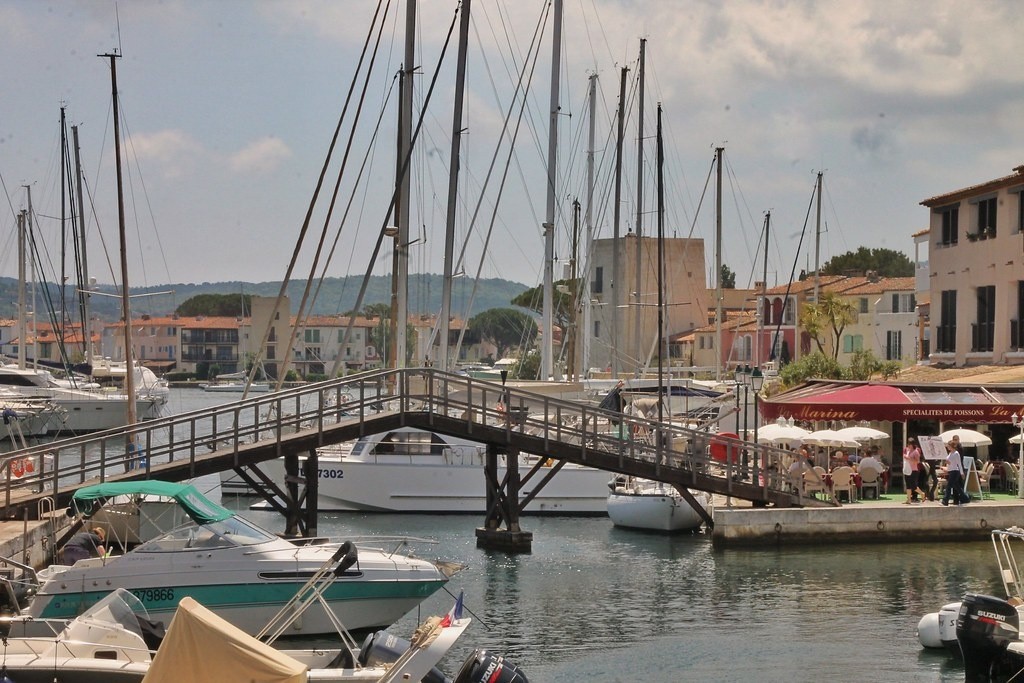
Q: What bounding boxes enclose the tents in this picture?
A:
[760,379,1024,493]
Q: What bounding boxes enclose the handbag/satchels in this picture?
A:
[917,462,930,476]
[903,451,912,475]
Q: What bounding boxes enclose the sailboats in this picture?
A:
[215,0,824,513]
[0,2,223,511]
[608,103,712,534]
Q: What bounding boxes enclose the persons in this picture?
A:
[903,435,971,506]
[779,443,890,502]
[62,527,106,567]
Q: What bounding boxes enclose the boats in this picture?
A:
[0,542,526,683]
[916,527,1024,683]
[200,372,270,392]
[0,480,448,634]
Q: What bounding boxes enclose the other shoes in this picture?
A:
[902,500,911,504]
[938,502,946,506]
[921,492,926,502]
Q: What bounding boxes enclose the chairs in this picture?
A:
[923,461,1019,500]
[790,462,887,503]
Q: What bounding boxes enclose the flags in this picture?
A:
[437,589,464,628]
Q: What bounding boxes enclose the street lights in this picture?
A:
[748,365,764,485]
[1011,412,1024,499]
[732,362,743,437]
[739,364,752,478]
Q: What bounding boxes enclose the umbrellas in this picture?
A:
[757,423,810,491]
[803,428,862,475]
[938,427,992,447]
[1008,433,1024,444]
[837,424,891,462]
